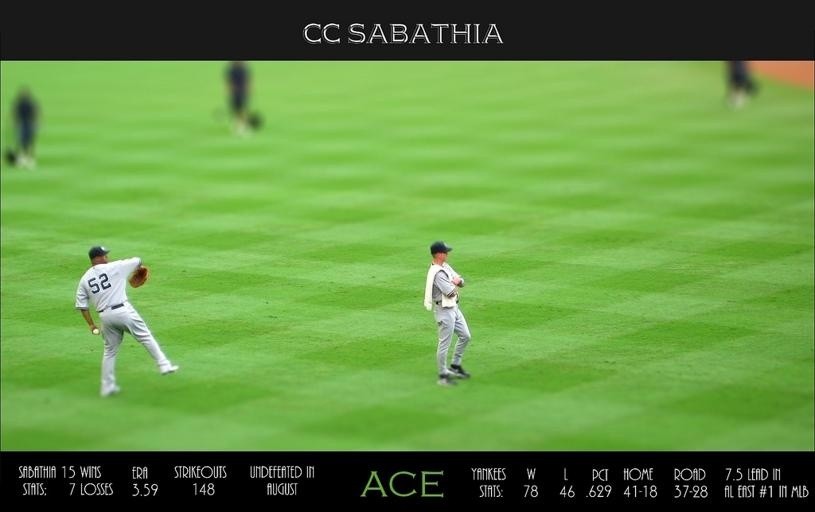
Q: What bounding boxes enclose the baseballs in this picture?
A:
[93,328,99,335]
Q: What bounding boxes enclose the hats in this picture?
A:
[430,241,452,254]
[88,246,110,260]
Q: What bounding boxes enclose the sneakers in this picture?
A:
[438,363,470,386]
[160,365,179,375]
[100,386,120,397]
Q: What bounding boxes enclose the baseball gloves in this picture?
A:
[129,265,148,288]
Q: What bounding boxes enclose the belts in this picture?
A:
[436,302,441,305]
[96,303,124,314]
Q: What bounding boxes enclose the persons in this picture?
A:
[221,60,250,138]
[11,85,41,170]
[423,242,471,389]
[724,60,752,112]
[74,246,179,399]
